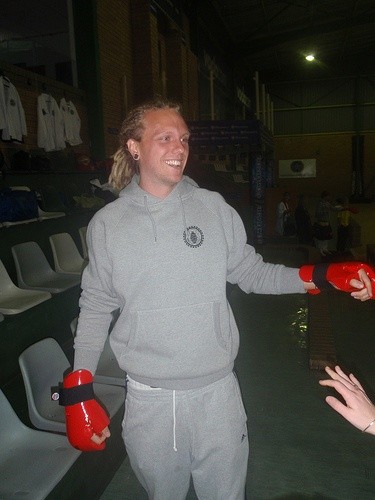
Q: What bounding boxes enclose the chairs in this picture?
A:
[0,186,126,500]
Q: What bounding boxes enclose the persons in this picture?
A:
[293,194,315,244]
[314,191,344,257]
[335,195,354,255]
[276,190,290,236]
[52,98,375,500]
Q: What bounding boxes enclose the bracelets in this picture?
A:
[361,419,375,434]
